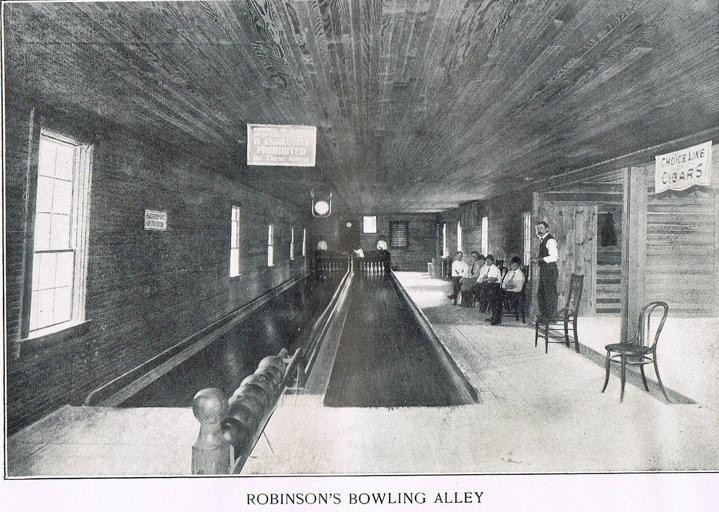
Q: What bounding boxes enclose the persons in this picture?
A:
[376,235,388,270]
[529,221,560,325]
[448,250,525,326]
[316,235,328,270]
[350,243,367,269]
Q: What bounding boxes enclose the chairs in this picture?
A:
[534,273,584,354]
[459,258,530,324]
[602,300,674,404]
